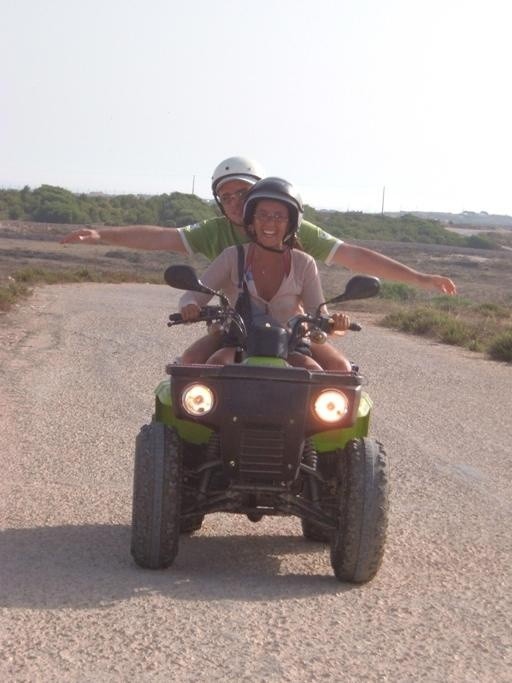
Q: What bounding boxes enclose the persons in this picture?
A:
[176,178,350,371]
[63,156,456,371]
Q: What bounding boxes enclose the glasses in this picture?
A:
[220,185,251,206]
[251,209,291,226]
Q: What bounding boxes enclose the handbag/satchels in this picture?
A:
[221,243,253,337]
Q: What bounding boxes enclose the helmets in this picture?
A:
[210,156,264,196]
[241,174,305,233]
